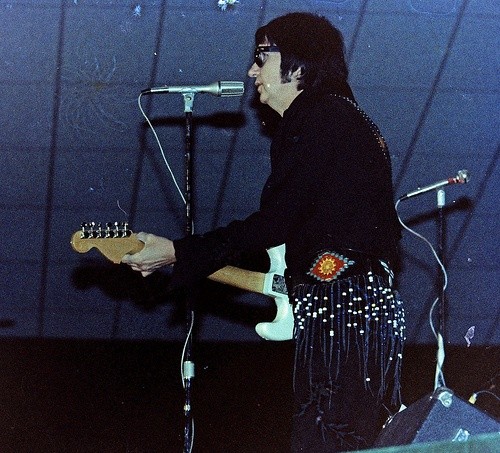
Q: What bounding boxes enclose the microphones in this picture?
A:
[399,169,471,201]
[182,361,194,418]
[141,80,245,98]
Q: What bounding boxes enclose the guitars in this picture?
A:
[71,221,297,340]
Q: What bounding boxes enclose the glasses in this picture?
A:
[254,46,280,67]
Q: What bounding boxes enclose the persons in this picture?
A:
[122,11,406,453]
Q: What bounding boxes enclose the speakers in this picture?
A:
[374,388,500,449]
